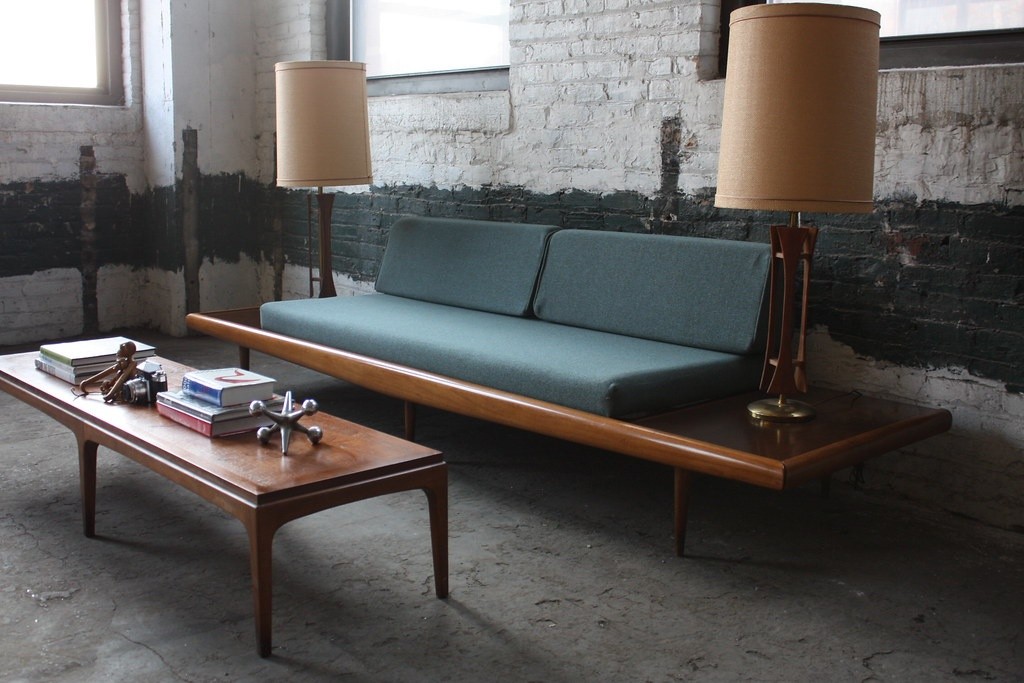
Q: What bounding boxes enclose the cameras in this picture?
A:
[120,360,168,405]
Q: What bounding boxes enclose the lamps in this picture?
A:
[275,61,373,298]
[713,4,881,423]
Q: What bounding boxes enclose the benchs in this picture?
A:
[184,205,952,558]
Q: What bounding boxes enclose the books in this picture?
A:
[155,367,297,441]
[34,335,158,386]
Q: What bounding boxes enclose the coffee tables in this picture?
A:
[0,350,449,657]
